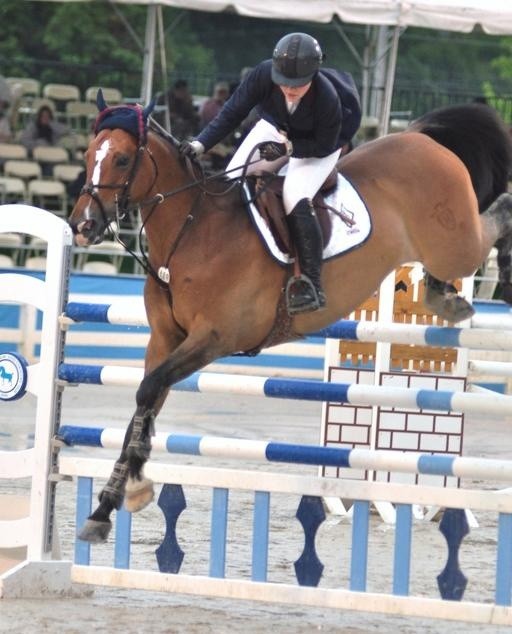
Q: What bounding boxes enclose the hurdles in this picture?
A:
[1,203,512,625]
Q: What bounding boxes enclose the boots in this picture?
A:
[285,198,327,307]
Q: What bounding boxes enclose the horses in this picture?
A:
[67,89,512,543]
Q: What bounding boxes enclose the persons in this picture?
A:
[1,90,13,141]
[180,30,364,320]
[200,83,231,143]
[235,62,260,133]
[156,79,196,133]
[20,104,67,149]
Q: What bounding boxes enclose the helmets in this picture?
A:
[271,33,322,86]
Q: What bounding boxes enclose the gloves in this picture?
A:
[259,142,286,161]
[176,141,204,158]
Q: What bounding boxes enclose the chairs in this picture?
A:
[0,77,126,275]
